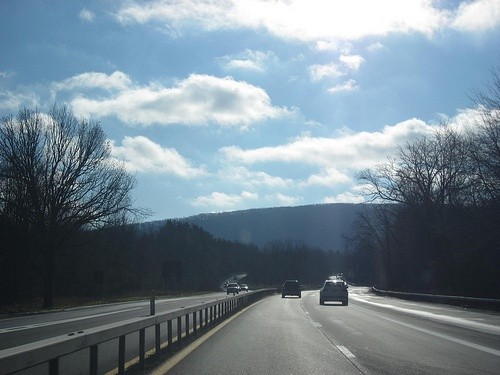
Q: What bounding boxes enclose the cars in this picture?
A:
[227,283,239,294]
[239,284,249,292]
[319,279,349,306]
[281,280,302,298]
